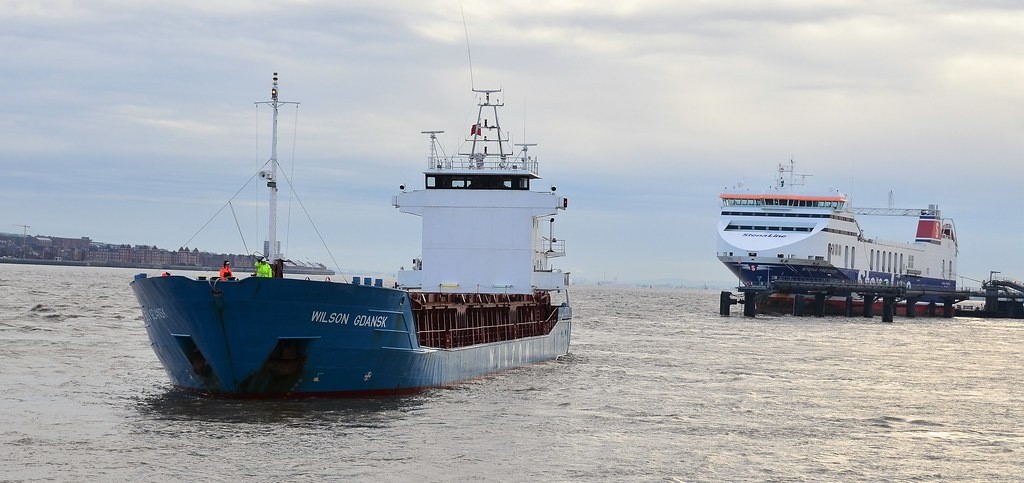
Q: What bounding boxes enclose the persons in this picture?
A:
[255,257,272,277]
[220,261,232,281]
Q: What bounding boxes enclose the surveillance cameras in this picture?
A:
[399,183,406,190]
[258,170,271,180]
[551,185,556,191]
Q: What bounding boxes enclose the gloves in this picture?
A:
[257,259,261,262]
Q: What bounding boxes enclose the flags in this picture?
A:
[471,124,481,136]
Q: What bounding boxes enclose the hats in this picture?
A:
[261,257,266,261]
[223,260,230,265]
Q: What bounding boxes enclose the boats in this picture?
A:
[716,155,958,318]
[131,0,573,409]
